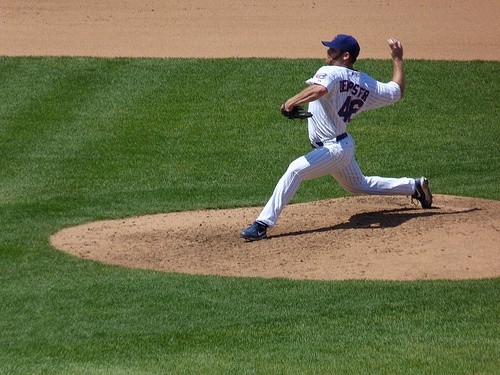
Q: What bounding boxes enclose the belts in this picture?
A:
[311,132,348,148]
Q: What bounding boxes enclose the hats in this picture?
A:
[322,33,360,57]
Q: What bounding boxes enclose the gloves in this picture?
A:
[282,105,312,118]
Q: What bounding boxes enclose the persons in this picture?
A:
[240,34,432,241]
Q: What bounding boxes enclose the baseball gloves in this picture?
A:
[281,103,313,119]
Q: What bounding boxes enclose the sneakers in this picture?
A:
[412,176,432,209]
[240,222,267,239]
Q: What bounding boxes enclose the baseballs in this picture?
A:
[387,38,393,44]
